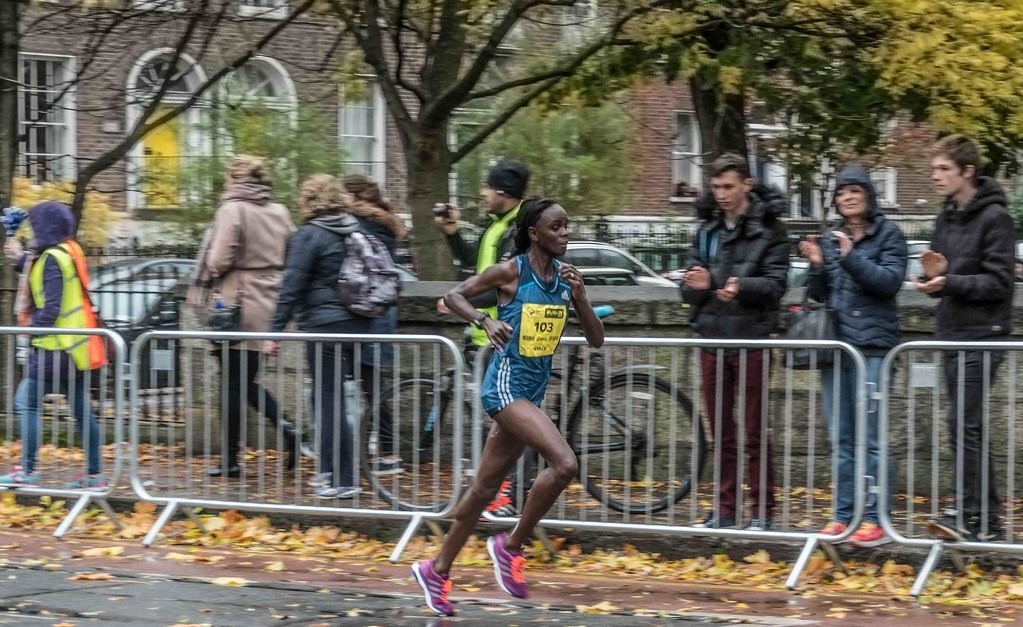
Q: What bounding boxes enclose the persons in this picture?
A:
[911,133,1016,543]
[410,195,605,618]
[796,168,909,547]
[262,171,408,499]
[679,153,791,530]
[435,157,532,520]
[0,201,110,492]
[185,152,308,477]
[300,175,405,475]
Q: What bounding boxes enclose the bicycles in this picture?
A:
[354,312,711,521]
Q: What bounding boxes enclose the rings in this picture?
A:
[570,272,575,278]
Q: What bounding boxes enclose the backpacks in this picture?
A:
[315,231,400,318]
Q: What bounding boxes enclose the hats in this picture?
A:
[489,158,529,200]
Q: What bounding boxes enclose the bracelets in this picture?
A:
[474,311,491,329]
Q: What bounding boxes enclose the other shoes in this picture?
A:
[283,426,307,470]
[209,462,239,477]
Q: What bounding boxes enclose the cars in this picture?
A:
[659,240,1023,317]
[560,234,680,291]
[80,255,204,393]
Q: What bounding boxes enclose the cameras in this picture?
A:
[433,203,450,219]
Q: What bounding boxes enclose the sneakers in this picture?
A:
[845,520,894,547]
[927,514,1004,545]
[313,485,362,499]
[367,459,403,476]
[487,532,529,601]
[412,557,455,616]
[300,441,316,460]
[65,473,106,490]
[0,466,41,488]
[307,472,334,487]
[820,522,847,544]
[746,517,769,534]
[479,481,530,523]
[694,512,733,529]
[941,504,957,516]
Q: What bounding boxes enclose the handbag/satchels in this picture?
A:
[209,302,240,346]
[781,278,837,369]
[91,307,115,364]
